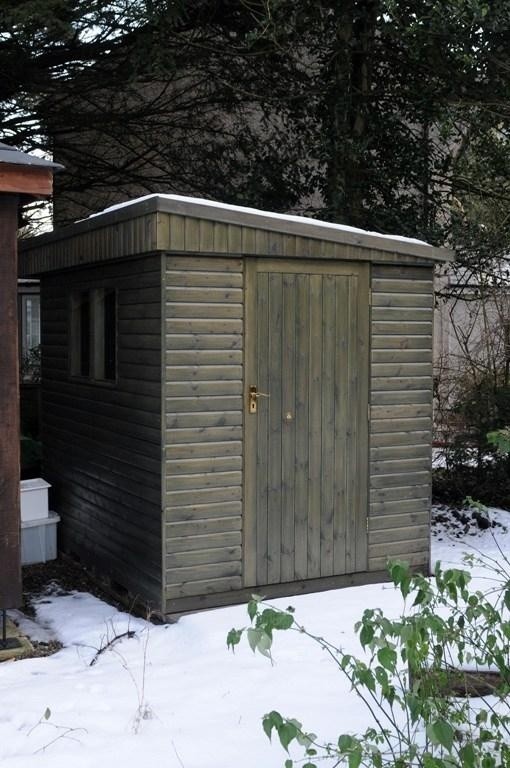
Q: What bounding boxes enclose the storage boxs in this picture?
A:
[21,513,61,565]
[20,478,52,522]
